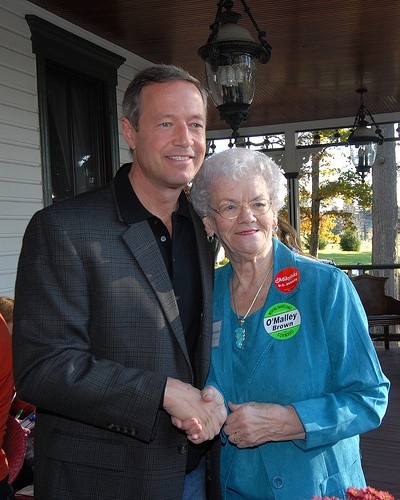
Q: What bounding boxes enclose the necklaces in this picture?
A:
[231,259,275,349]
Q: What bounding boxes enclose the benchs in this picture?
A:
[334,264,400,350]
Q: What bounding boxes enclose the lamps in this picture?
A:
[197,0,271,139]
[346,88,384,184]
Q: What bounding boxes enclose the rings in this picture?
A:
[233,432,240,444]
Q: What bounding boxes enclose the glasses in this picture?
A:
[207,198,273,220]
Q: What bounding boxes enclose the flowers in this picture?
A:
[311,485,400,500]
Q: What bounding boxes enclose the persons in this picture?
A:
[10,63,226,500]
[170,147,391,499]
